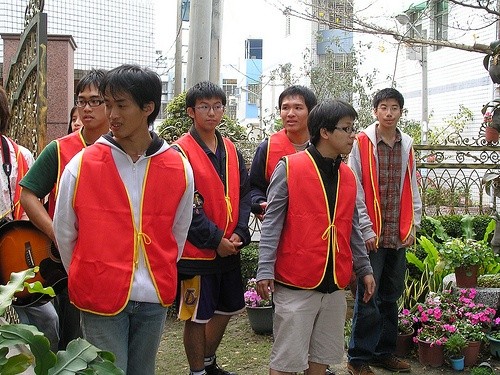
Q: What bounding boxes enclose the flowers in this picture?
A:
[399,288,500,347]
[244,278,272,307]
[483,112,492,127]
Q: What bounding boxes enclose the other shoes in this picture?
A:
[188,358,238,375]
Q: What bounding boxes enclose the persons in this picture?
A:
[255,100,376,375]
[347,88,422,375]
[19,68,110,349]
[170,80,251,375]
[0,87,58,350]
[250,86,320,224]
[52,64,194,375]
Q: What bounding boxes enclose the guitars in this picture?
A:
[1,220,70,310]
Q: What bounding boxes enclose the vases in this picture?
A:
[461,341,480,367]
[486,127,499,142]
[415,336,444,368]
[245,305,273,336]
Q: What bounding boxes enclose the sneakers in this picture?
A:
[347,358,377,375]
[369,353,411,372]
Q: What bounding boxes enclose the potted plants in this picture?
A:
[439,238,500,288]
[398,317,414,356]
[487,331,500,357]
[445,333,470,370]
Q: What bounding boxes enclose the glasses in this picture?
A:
[327,125,357,134]
[376,105,400,113]
[191,105,224,111]
[74,99,106,107]
